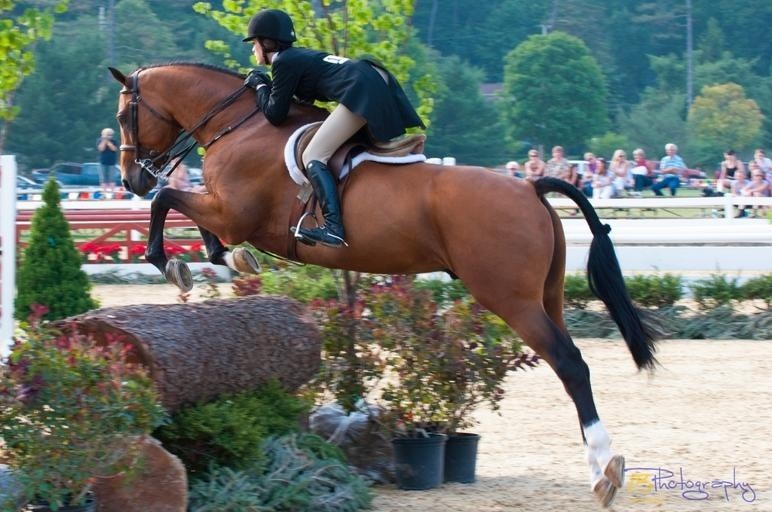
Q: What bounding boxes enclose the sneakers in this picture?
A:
[733,209,757,218]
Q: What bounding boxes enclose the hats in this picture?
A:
[506,161,519,170]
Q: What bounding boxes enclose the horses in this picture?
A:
[107,58,666,509]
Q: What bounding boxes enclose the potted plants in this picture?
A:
[0,301,174,512]
[316,272,544,490]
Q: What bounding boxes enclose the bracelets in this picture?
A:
[107,141,111,146]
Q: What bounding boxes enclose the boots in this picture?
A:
[290,161,345,247]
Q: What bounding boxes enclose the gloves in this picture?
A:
[245,70,273,89]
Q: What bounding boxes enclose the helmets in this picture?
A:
[242,9,296,41]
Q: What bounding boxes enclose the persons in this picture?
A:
[242,8,426,249]
[94,127,118,192]
[168,157,191,193]
[499,140,772,217]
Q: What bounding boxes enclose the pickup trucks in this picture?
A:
[51,161,123,186]
